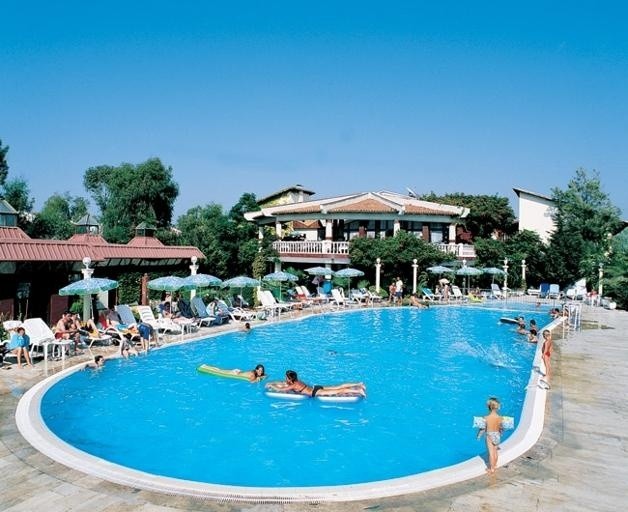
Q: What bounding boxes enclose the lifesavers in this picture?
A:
[501,416,514,431]
[472,417,487,430]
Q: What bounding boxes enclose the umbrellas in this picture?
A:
[184,273,222,286]
[59,278,119,304]
[333,267,365,301]
[262,271,299,302]
[426,266,505,276]
[146,275,196,302]
[304,266,336,297]
[220,274,261,308]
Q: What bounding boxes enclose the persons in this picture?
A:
[542,330,551,376]
[162,302,181,319]
[265,370,366,399]
[56,304,154,371]
[200,364,264,381]
[10,327,32,368]
[388,277,403,303]
[517,302,569,342]
[477,398,504,474]
[243,323,250,332]
[438,278,450,301]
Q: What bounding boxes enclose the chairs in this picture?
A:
[1,320,24,358]
[420,283,524,302]
[528,282,618,309]
[94,322,128,342]
[71,314,112,348]
[115,304,154,345]
[137,305,181,335]
[158,285,382,337]
[24,318,72,362]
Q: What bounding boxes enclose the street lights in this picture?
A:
[80,257,94,326]
[520,259,527,289]
[461,258,467,295]
[597,262,605,296]
[501,260,509,297]
[374,257,381,294]
[410,258,419,294]
[189,255,200,302]
